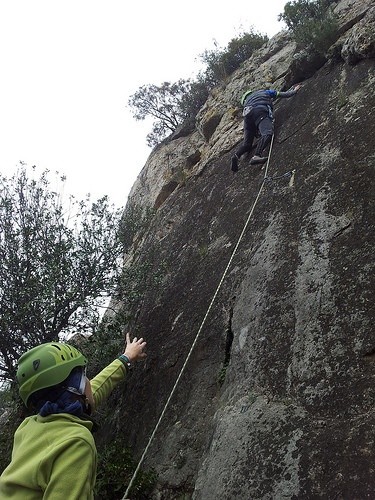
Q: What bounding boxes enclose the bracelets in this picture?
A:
[121,355,130,366]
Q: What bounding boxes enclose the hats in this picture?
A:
[30,367,87,411]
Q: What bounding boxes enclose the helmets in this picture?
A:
[15,342,88,408]
[240,91,252,106]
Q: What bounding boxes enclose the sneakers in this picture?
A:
[231,154,239,172]
[249,156,267,164]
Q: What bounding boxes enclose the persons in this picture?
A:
[0,332,147,500]
[230,85,301,172]
